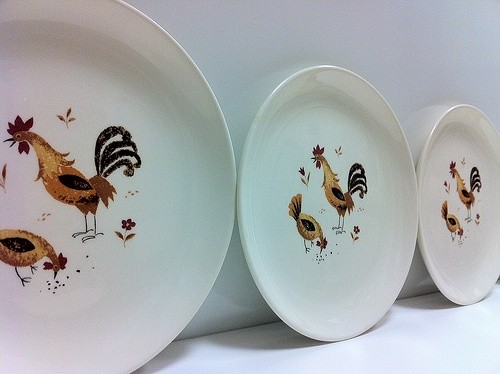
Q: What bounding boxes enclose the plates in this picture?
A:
[237,63,419,341]
[416,104,500,306]
[0,2,235,373]
